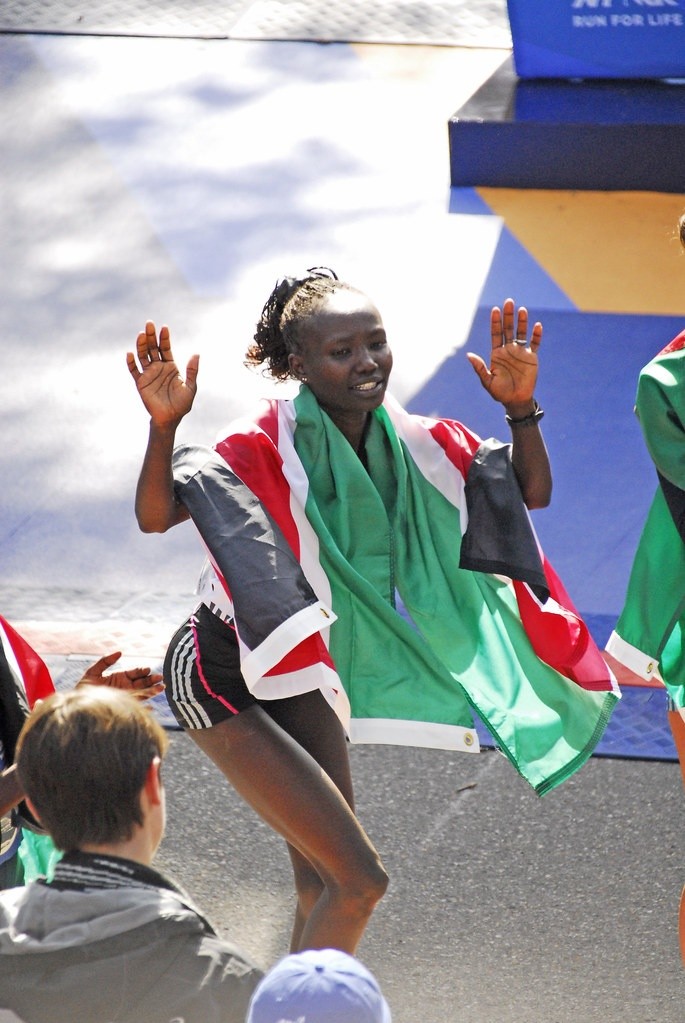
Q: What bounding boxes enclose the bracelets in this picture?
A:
[507,399,544,432]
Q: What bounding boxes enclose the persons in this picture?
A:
[0,618,394,1022]
[127,264,555,960]
[608,209,685,980]
